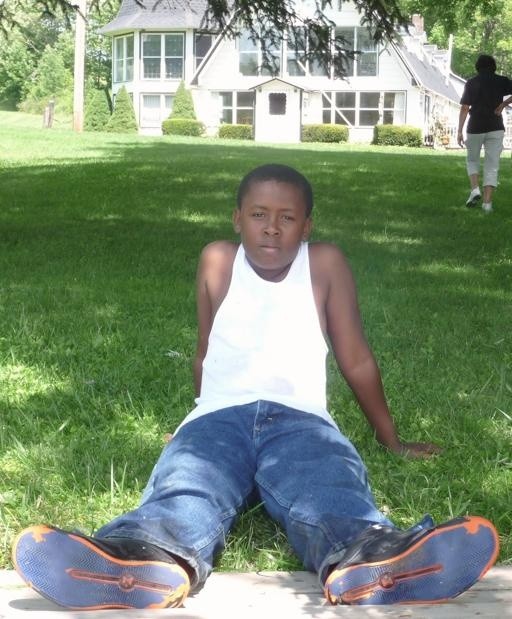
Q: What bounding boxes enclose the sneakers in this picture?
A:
[13,524,191,611]
[482,202,492,211]
[324,514,498,605]
[465,186,481,207]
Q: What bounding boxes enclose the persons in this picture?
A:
[6,160,506,613]
[456,54,512,212]
